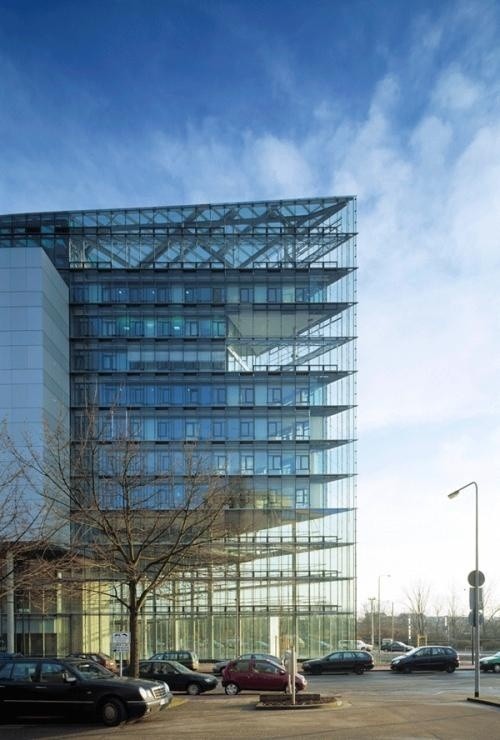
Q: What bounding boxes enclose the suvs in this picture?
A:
[301,650,375,675]
[148,649,199,671]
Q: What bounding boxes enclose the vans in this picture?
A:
[0,655,175,728]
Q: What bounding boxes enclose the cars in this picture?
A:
[389,642,461,677]
[112,633,334,654]
[221,657,307,695]
[338,639,374,651]
[212,653,282,677]
[71,652,116,673]
[115,659,219,696]
[479,649,500,674]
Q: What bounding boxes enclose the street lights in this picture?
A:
[444,480,480,698]
[378,573,392,650]
